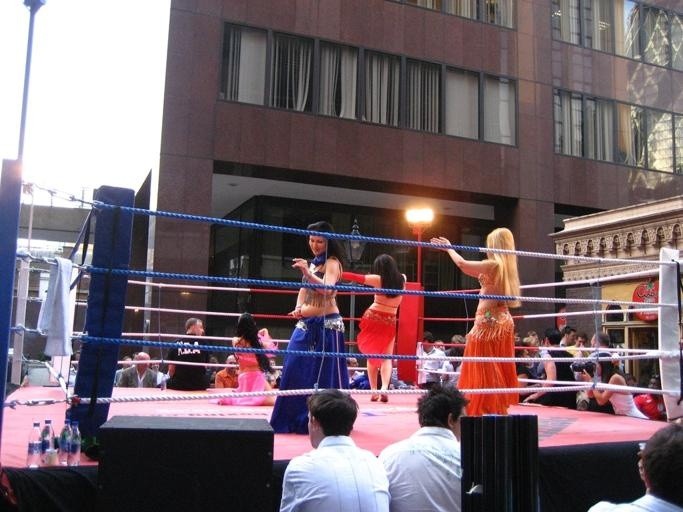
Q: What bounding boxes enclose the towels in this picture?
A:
[39,258,74,357]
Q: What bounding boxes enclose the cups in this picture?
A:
[42,448,59,465]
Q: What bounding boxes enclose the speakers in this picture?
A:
[461,416,538,512]
[97,416,274,512]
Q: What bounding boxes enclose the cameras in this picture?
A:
[573,362,596,377]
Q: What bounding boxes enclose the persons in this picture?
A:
[582,423,683,512]
[66,223,669,431]
[280,389,392,512]
[376,383,473,508]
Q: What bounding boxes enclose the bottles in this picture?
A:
[41,418,54,462]
[67,422,81,465]
[161,374,166,390]
[58,419,71,464]
[25,422,42,469]
